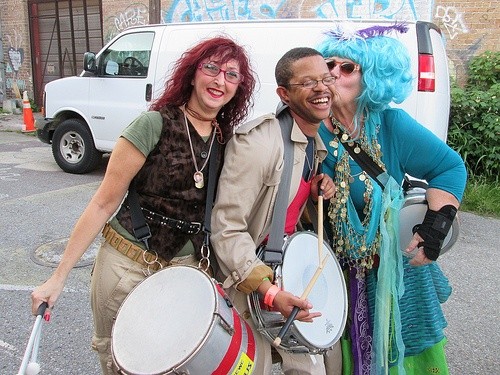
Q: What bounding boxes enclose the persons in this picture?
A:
[31,38,256,375]
[317,35,467,375]
[211,47,344,375]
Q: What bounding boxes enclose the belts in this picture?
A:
[102,223,206,271]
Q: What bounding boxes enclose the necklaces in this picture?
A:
[328,110,385,277]
[183,103,217,189]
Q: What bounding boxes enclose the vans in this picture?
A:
[37,19,451,176]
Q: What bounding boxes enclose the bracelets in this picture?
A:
[265,285,281,308]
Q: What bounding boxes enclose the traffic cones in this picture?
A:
[21,90,36,133]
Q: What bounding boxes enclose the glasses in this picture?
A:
[197,63,245,84]
[284,76,336,89]
[324,59,361,75]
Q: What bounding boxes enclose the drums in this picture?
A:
[246,227,352,361]
[399,180,460,259]
[108,259,257,375]
